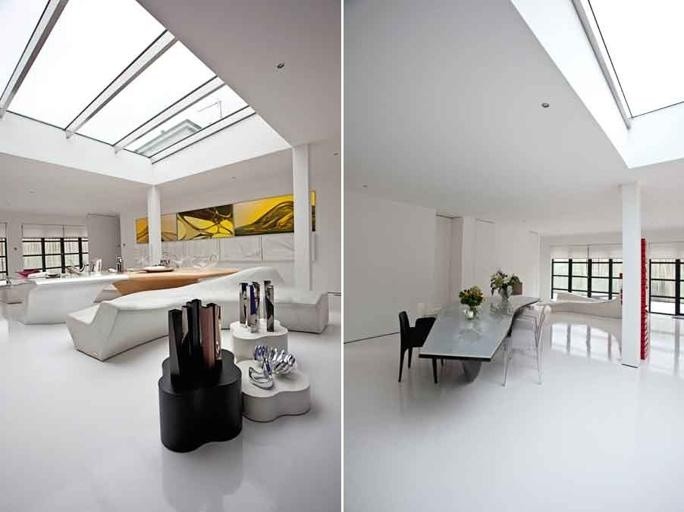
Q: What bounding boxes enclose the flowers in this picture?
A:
[488,270,521,296]
[458,285,485,312]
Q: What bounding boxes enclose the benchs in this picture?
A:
[548,291,621,321]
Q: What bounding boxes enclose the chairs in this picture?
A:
[397,301,546,386]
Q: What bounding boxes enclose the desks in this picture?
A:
[418,297,541,383]
